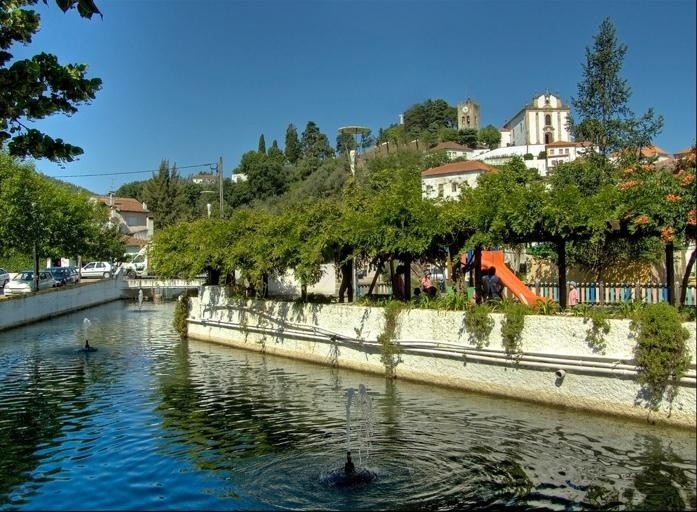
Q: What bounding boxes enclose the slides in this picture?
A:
[480,251,552,306]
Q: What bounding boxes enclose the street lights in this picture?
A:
[338,126,371,303]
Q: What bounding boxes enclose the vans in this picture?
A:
[120,244,148,279]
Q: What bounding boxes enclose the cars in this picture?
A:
[0,266,81,296]
[76,261,113,279]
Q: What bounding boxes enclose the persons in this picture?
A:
[482,266,502,301]
[392,264,406,302]
[421,272,436,298]
[568,282,578,310]
[412,288,421,299]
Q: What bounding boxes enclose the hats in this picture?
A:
[569,281,576,288]
